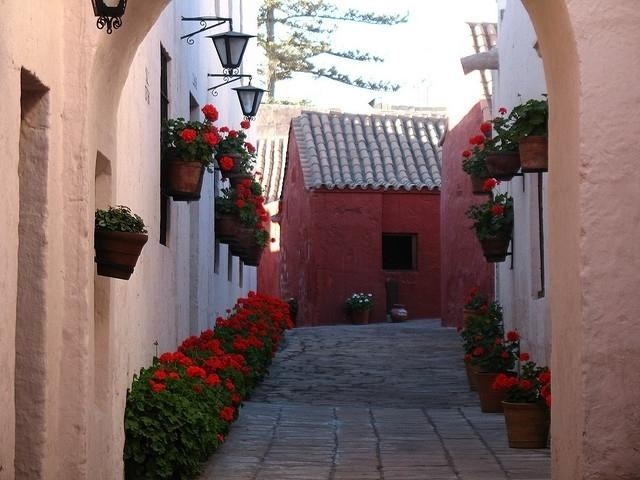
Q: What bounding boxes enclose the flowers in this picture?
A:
[459,287,556,406]
[464,177,516,239]
[164,103,223,162]
[345,291,375,311]
[215,123,275,247]
[460,108,517,176]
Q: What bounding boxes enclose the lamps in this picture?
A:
[180,15,271,122]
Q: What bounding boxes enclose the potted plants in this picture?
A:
[93,204,149,281]
[507,95,550,173]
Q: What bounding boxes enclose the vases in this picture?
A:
[216,208,264,267]
[477,371,552,449]
[479,234,508,264]
[350,310,369,325]
[463,151,518,192]
[165,160,206,203]
